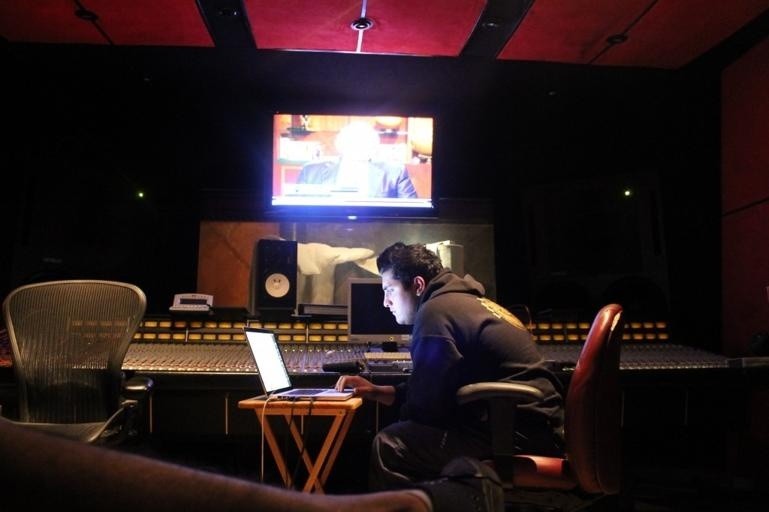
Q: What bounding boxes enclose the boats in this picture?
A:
[249,238,297,312]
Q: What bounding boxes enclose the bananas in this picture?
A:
[363,350,413,362]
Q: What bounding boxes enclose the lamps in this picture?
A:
[456,303,624,512]
[4,279,153,445]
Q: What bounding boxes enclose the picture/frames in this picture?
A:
[237,392,364,496]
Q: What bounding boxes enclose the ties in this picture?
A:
[349,277,414,346]
[269,108,438,222]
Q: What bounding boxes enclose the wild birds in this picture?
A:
[242,326,355,402]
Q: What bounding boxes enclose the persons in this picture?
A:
[333,241,564,493]
[296,123,420,198]
